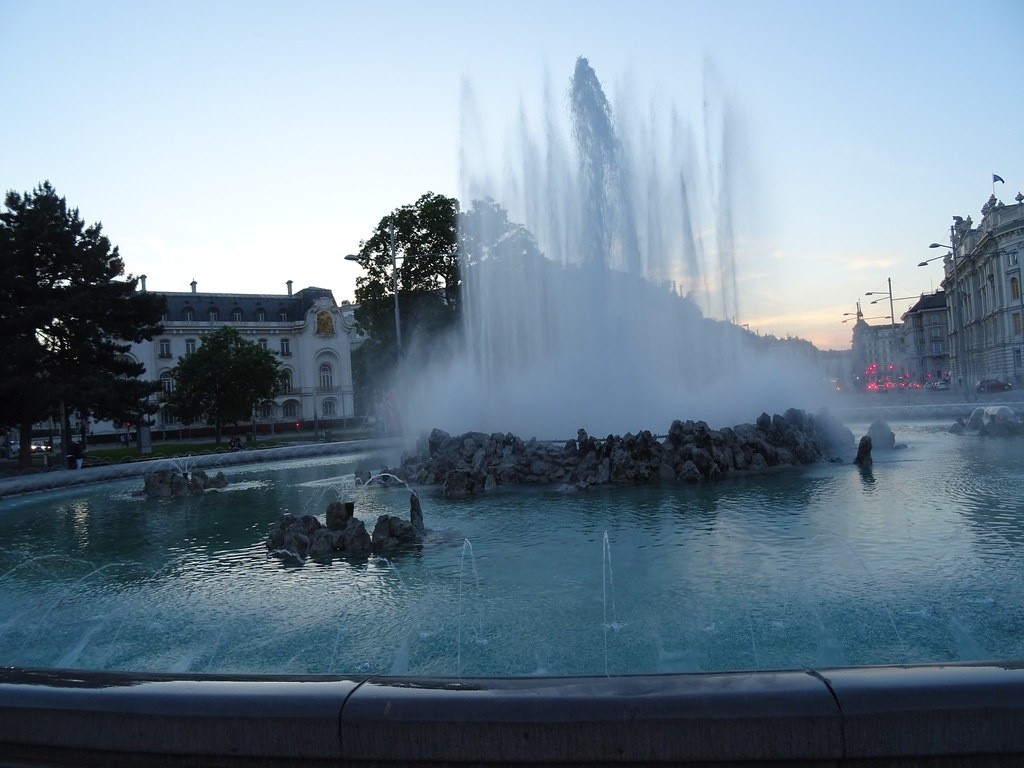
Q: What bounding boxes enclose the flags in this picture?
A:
[994,175,1004,184]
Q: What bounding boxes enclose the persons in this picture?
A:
[121,434,132,447]
[229,436,242,452]
[43,440,48,446]
[959,377,962,386]
[66,430,84,470]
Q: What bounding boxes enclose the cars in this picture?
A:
[923,382,947,389]
[31,442,51,452]
[976,379,1012,393]
[9,440,22,454]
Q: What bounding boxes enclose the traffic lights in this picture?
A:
[125,421,132,427]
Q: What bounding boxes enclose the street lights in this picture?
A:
[917,225,968,390]
[342,222,459,431]
[843,302,892,370]
[864,278,900,382]
